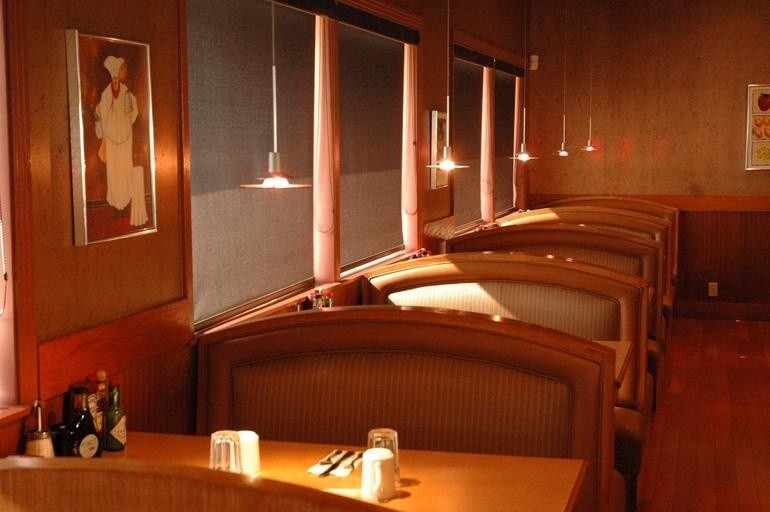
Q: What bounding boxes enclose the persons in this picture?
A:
[93,54,138,210]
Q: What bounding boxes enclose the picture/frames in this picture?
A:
[744,82,770,171]
[431,110,450,190]
[66,28,157,246]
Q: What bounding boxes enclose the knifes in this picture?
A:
[319,451,354,477]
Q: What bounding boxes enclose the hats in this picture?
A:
[104,56,123,78]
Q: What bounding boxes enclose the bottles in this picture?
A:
[23,370,127,458]
[295,289,333,311]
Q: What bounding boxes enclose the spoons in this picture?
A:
[320,450,342,466]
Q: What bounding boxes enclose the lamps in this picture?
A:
[427,0,470,171]
[239,0,313,189]
[508,1,538,162]
[580,54,598,152]
[553,50,569,157]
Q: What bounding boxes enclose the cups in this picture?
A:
[208,430,241,470]
[368,428,400,479]
[361,447,395,503]
[235,429,261,472]
